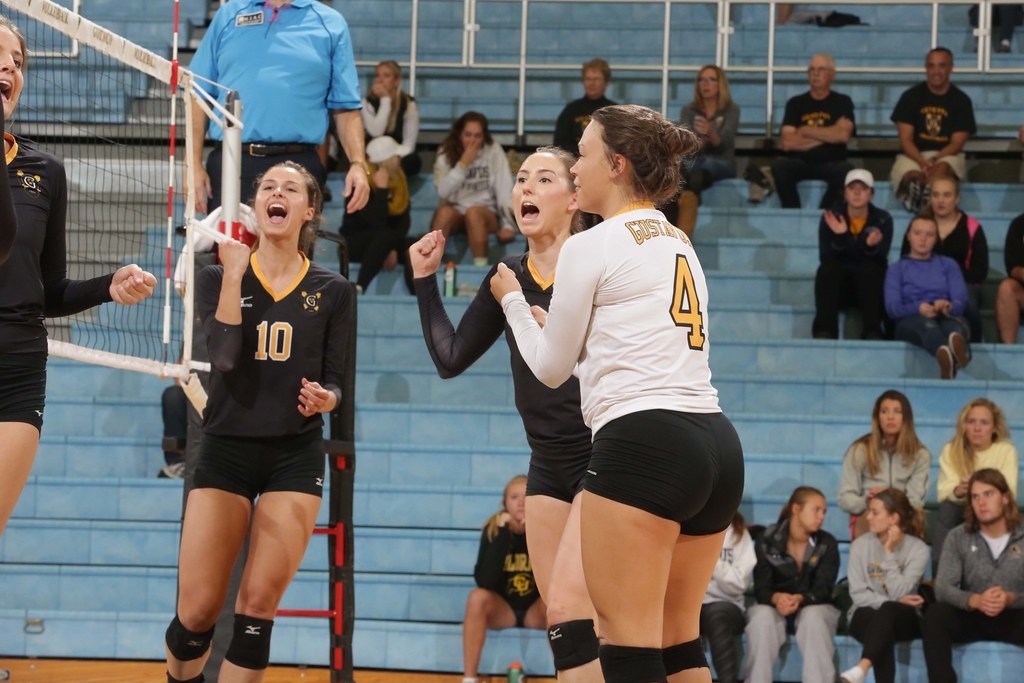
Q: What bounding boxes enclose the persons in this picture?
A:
[0,13,158,541]
[656,65,740,239]
[769,55,857,209]
[336,59,422,296]
[699,389,1024,683]
[810,169,1024,380]
[969,3,1024,53]
[164,161,352,683]
[461,474,546,683]
[156,311,212,478]
[889,46,977,215]
[553,58,619,230]
[182,0,371,262]
[776,4,794,24]
[428,110,519,266]
[409,103,745,683]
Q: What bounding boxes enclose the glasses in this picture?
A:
[699,78,717,83]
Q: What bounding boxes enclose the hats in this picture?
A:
[366,136,411,163]
[845,168,874,188]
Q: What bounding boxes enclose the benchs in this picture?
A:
[0,1,1024,683]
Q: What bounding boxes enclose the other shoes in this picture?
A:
[840,666,864,683]
[949,331,969,368]
[936,345,956,379]
[903,177,921,212]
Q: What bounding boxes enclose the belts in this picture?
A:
[241,144,311,157]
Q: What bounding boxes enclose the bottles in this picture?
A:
[507,660,525,683]
[442,261,458,297]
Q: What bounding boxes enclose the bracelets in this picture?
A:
[350,161,370,178]
